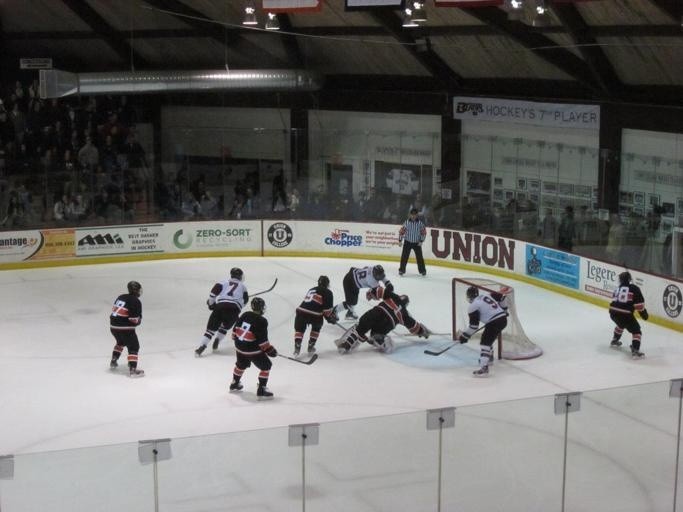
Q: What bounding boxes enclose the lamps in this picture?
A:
[413,3,427,22]
[242,5,258,25]
[508,6,524,21]
[264,14,281,29]
[531,8,551,27]
[402,8,419,28]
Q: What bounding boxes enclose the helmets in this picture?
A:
[249,298,264,311]
[465,285,479,299]
[400,294,410,305]
[373,265,384,276]
[229,267,243,279]
[619,272,633,284]
[127,280,141,292]
[316,275,330,287]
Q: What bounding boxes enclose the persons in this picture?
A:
[194,267,248,356]
[333,265,394,319]
[398,209,427,275]
[408,191,426,227]
[609,272,649,360]
[427,191,673,275]
[229,297,277,397]
[109,281,145,377]
[292,275,339,356]
[337,287,429,353]
[459,286,510,375]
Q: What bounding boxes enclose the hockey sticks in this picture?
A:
[423,310,508,357]
[329,313,382,351]
[261,348,321,367]
[210,277,279,303]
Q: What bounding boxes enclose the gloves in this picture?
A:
[208,305,214,310]
[419,328,430,340]
[397,241,404,248]
[417,241,422,248]
[458,332,470,346]
[266,346,277,358]
[329,316,338,327]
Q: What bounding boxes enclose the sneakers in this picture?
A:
[229,384,243,390]
[194,349,202,355]
[333,309,339,321]
[611,338,623,346]
[130,370,144,374]
[345,311,358,318]
[212,342,219,349]
[630,347,644,355]
[421,270,427,277]
[257,388,273,396]
[398,268,405,275]
[110,360,118,367]
[473,366,489,374]
[308,347,315,353]
[337,342,351,351]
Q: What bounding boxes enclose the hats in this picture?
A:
[410,208,419,216]
